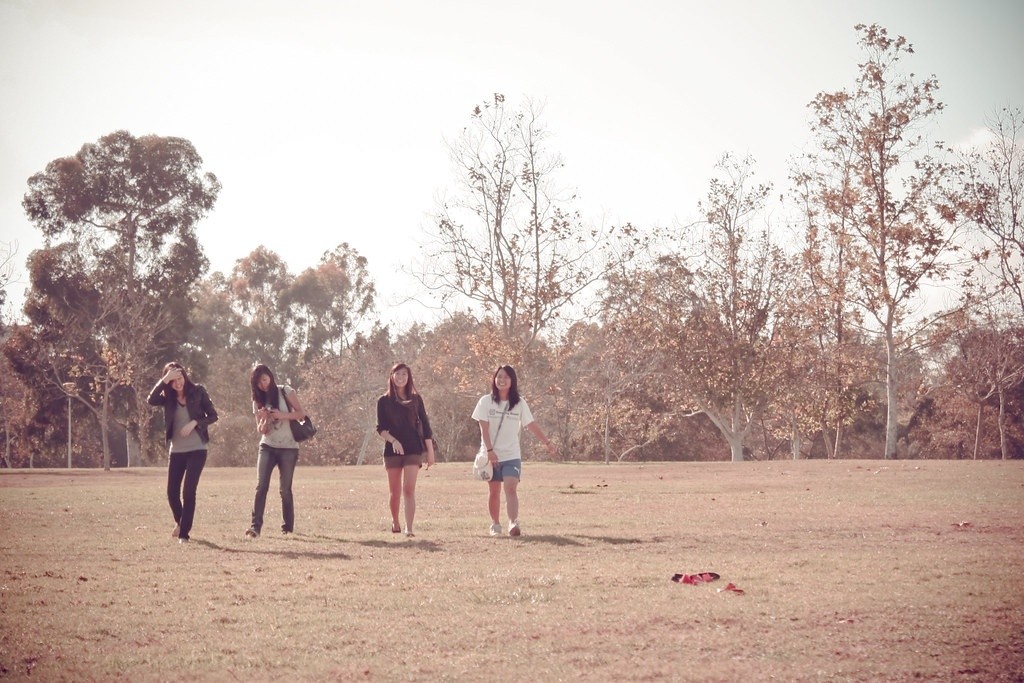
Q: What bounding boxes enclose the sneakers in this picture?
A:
[508,524,520,536]
[490,523,502,538]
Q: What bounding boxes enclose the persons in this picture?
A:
[245,365,306,538]
[470,365,555,536]
[376,362,435,537]
[146,361,218,544]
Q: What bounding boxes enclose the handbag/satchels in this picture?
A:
[414,392,439,453]
[282,384,318,442]
[473,452,493,481]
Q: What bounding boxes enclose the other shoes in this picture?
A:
[172,522,181,537]
[179,538,188,544]
[245,530,256,539]
[392,524,401,533]
[404,528,415,538]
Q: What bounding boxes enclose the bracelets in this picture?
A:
[546,441,550,445]
[392,439,397,443]
[488,449,493,452]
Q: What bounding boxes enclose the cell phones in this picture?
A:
[426,464,429,471]
